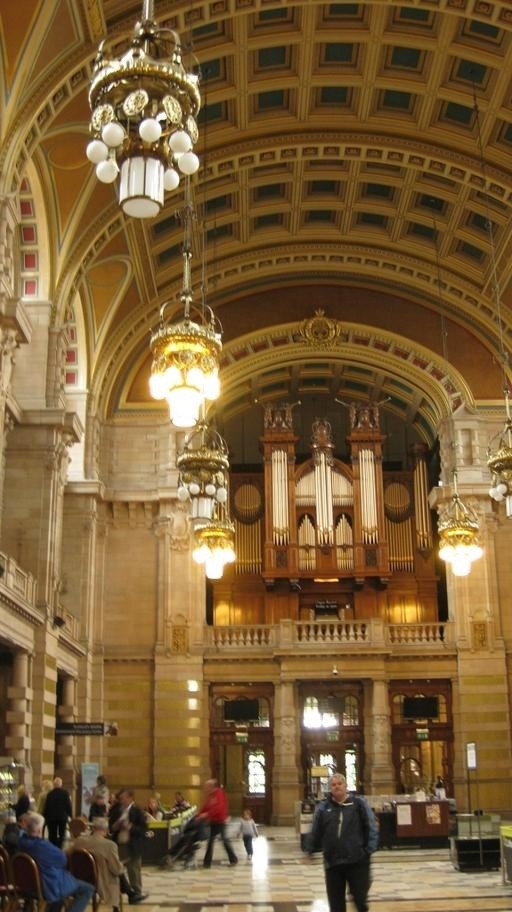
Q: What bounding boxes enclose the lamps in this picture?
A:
[193,522,236,579]
[311,766,329,799]
[176,401,230,525]
[427,195,483,576]
[147,175,223,429]
[86,1,203,218]
[468,69,512,519]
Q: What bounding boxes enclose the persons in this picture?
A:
[236,809,259,860]
[0,775,191,912]
[196,778,238,868]
[304,772,378,912]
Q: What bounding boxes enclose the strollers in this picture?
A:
[161,814,209,871]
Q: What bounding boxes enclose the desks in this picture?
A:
[500,825,512,885]
[142,805,196,866]
[448,812,511,872]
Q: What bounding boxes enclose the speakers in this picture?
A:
[404,698,437,718]
[224,700,259,720]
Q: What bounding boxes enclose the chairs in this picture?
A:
[0,856,32,912]
[69,847,124,912]
[70,819,87,839]
[8,852,74,912]
[0,844,35,911]
